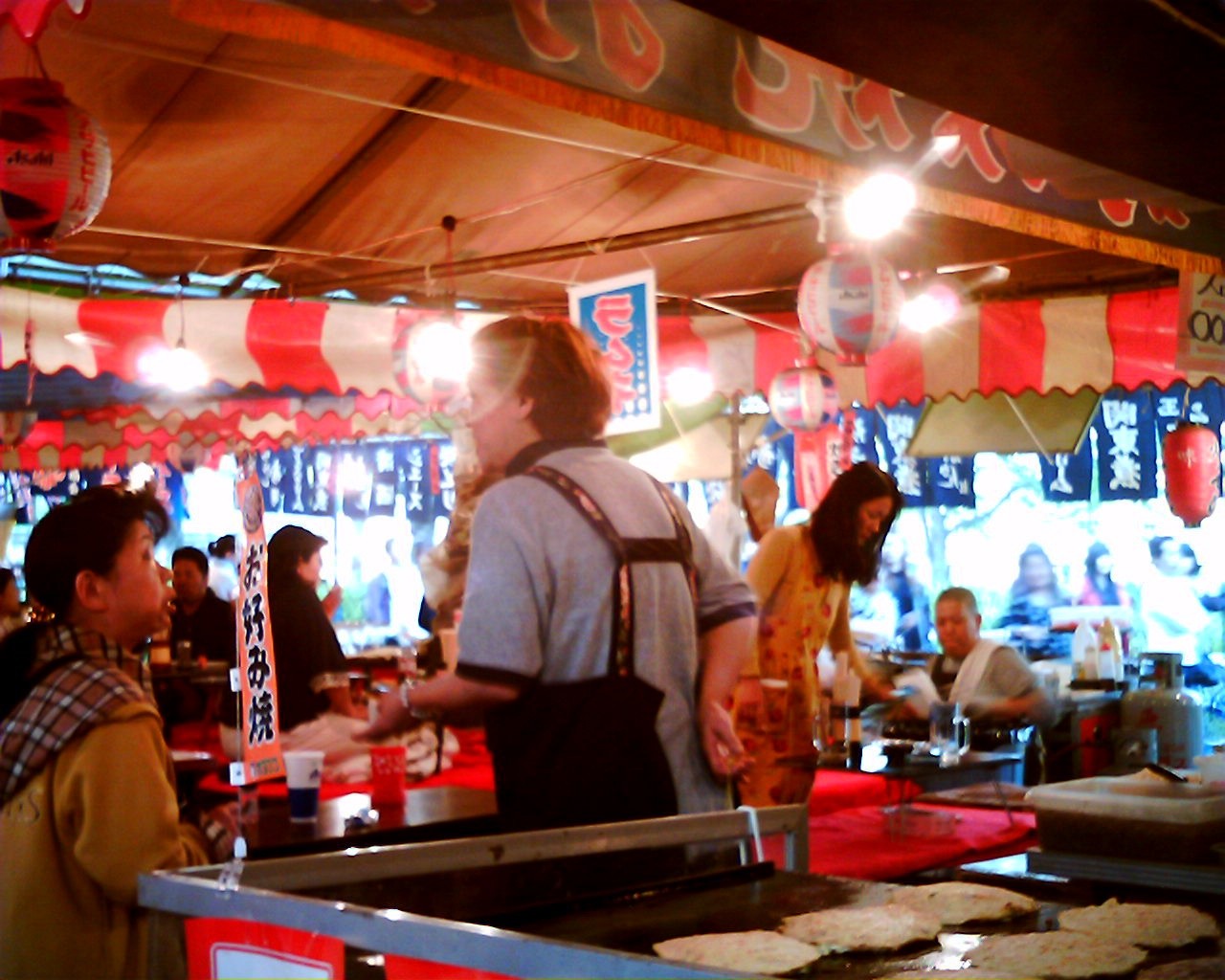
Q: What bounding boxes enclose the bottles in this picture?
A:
[1070,617,1124,684]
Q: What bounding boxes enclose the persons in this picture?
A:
[0,461,1225,980]
[350,312,762,898]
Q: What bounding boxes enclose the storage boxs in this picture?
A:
[1021,775,1225,871]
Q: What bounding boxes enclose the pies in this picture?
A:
[654,881,1221,980]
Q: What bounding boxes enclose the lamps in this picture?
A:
[154,211,470,391]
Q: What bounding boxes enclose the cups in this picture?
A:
[177,643,193,669]
[928,701,972,758]
[813,702,851,770]
[148,627,171,674]
[282,752,325,823]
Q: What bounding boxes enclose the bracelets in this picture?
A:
[398,680,434,719]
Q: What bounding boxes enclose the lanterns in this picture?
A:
[1166,417,1222,527]
[796,237,901,367]
[770,364,837,436]
[2,78,111,404]
